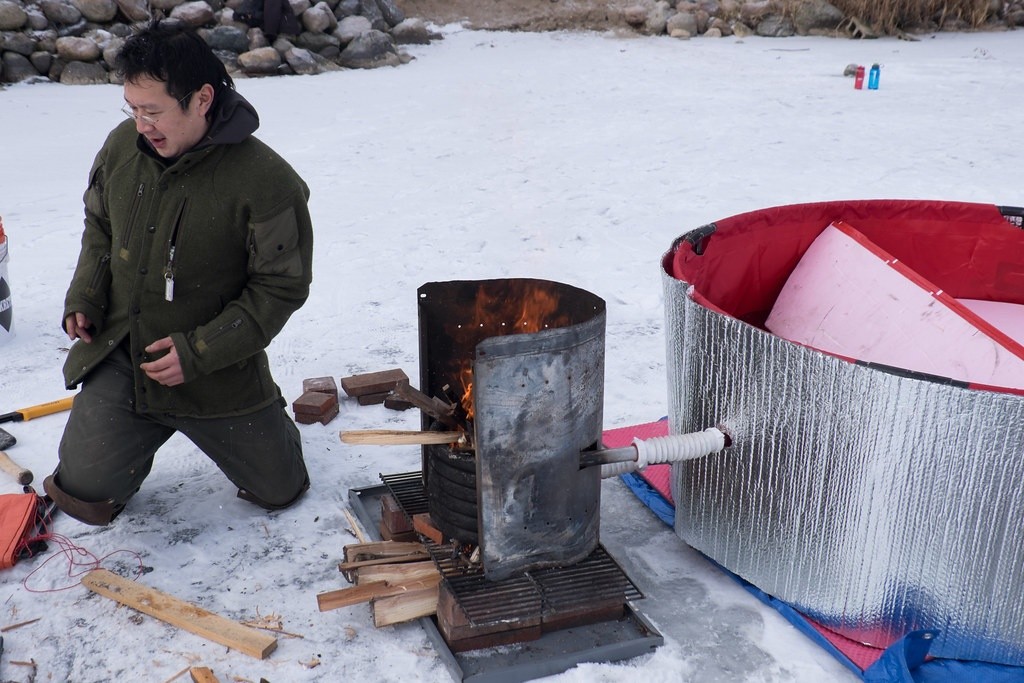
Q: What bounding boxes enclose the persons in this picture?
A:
[44,22,313,525]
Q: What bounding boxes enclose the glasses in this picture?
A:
[120,89,193,126]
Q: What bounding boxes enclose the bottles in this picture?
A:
[868,64,880,90]
[854,65,865,90]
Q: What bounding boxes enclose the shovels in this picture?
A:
[0,427,34,484]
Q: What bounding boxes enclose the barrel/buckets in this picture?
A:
[0,216,16,347]
